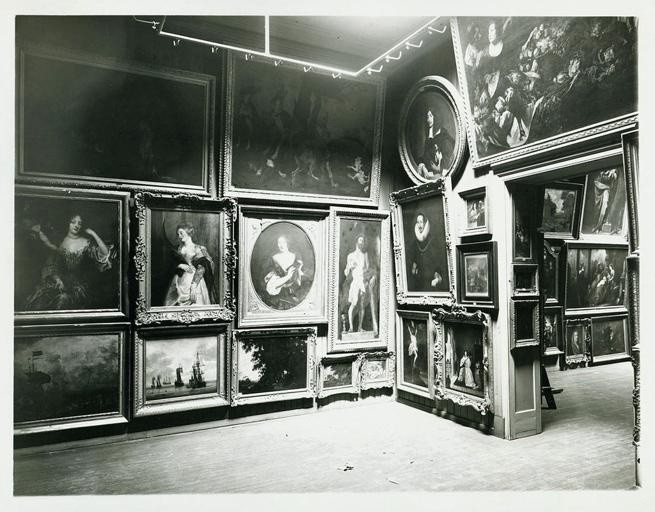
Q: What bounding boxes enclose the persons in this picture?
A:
[164,220,214,305]
[410,212,447,291]
[343,233,371,333]
[26,212,117,310]
[406,321,419,368]
[264,235,304,309]
[464,16,512,73]
[420,104,453,173]
[458,350,477,388]
[568,331,580,355]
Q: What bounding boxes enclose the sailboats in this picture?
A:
[150,353,207,390]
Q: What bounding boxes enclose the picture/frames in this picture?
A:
[509,264,541,297]
[543,240,559,303]
[458,185,494,239]
[562,317,592,366]
[389,177,456,307]
[17,40,218,197]
[223,46,387,208]
[541,305,566,357]
[13,185,130,320]
[433,307,496,418]
[359,352,397,393]
[449,16,639,169]
[134,320,233,420]
[396,309,435,401]
[131,193,236,323]
[326,206,395,352]
[623,255,640,445]
[535,179,585,239]
[13,322,133,445]
[576,167,628,239]
[620,128,640,253]
[315,355,360,400]
[558,240,630,313]
[455,240,500,312]
[510,299,541,352]
[512,183,536,261]
[230,325,319,408]
[236,207,331,325]
[591,313,633,365]
[396,75,469,188]
